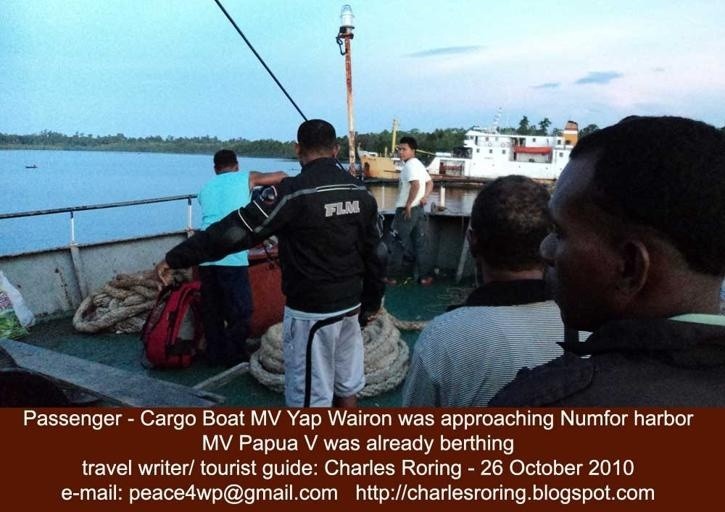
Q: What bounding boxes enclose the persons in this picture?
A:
[156,117,390,409]
[195,146,289,367]
[486,113,725,411]
[400,173,594,408]
[382,136,436,288]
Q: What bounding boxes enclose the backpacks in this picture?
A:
[139,280,203,372]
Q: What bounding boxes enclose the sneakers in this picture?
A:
[383,276,433,286]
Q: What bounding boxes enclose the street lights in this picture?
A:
[336,3,360,176]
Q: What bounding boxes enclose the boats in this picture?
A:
[355,111,582,192]
[24,165,38,168]
